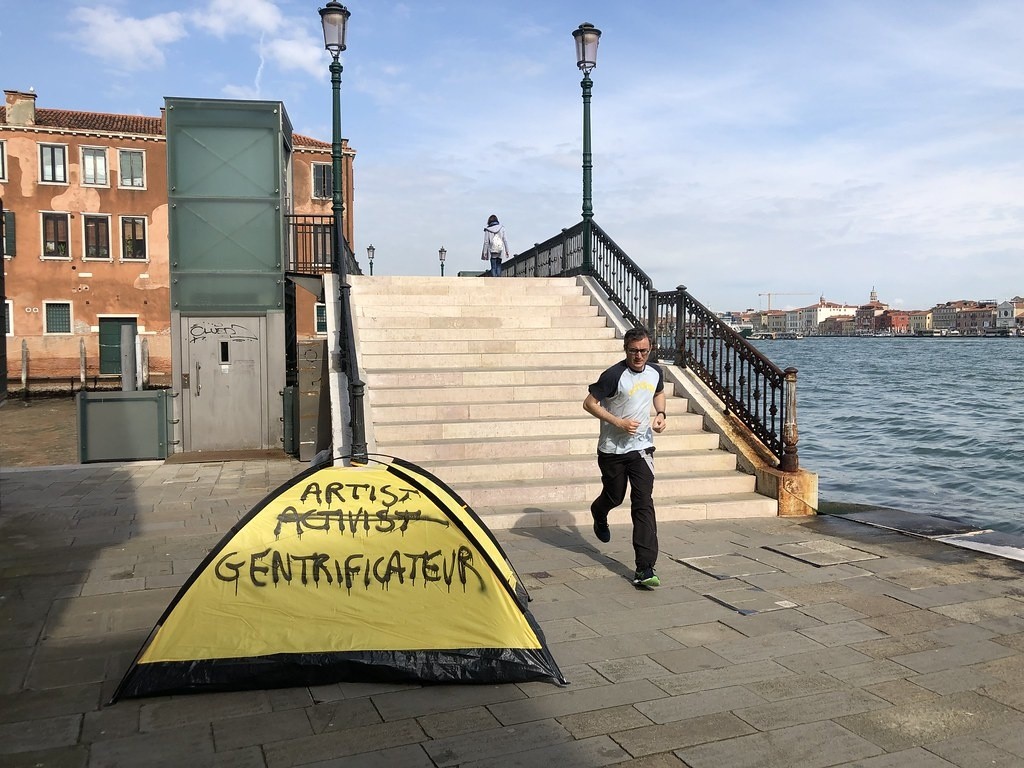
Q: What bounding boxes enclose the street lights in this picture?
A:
[365,244,375,275]
[316,1,352,274]
[572,22,605,273]
[438,246,448,276]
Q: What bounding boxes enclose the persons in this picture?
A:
[583,327,668,586]
[482,215,509,277]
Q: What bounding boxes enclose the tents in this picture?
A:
[100,453,572,707]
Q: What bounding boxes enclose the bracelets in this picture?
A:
[657,411,666,419]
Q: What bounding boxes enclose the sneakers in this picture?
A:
[634,567,661,587]
[591,505,611,542]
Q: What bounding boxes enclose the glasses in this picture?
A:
[626,345,651,355]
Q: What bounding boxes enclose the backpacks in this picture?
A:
[484,225,504,254]
